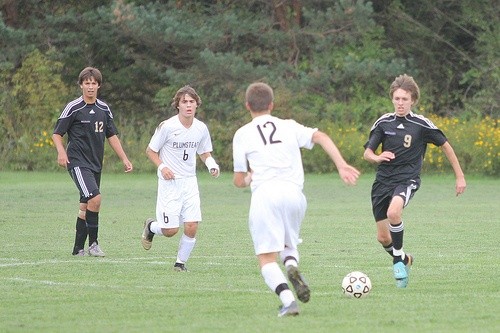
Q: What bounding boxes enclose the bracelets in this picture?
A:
[158,163,166,172]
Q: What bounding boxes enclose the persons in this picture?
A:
[141,84,221,274]
[362,73,467,289]
[231,82,361,318]
[51,66,133,258]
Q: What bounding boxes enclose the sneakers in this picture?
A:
[88,242,105,256]
[74,249,89,256]
[172,263,189,273]
[278,300,298,317]
[142,218,155,250]
[397,255,412,288]
[394,262,408,280]
[287,266,310,302]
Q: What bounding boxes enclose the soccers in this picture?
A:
[341,270,372,299]
